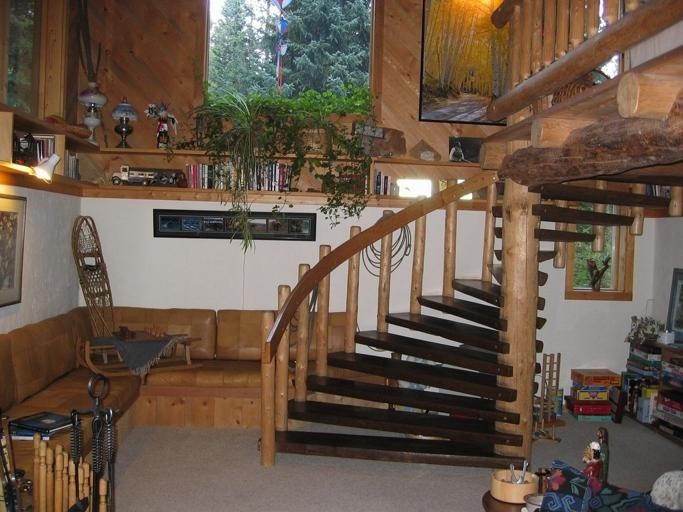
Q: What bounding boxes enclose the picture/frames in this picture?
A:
[417,0,509,126]
[665,267,682,344]
[152,209,316,241]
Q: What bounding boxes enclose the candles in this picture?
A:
[0,193,27,308]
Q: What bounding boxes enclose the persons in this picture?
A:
[450,141,464,161]
[579,441,604,497]
[594,426,611,483]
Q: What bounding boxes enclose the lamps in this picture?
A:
[31,153,60,185]
[111,96,138,148]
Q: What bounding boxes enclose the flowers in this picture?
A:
[622,315,666,343]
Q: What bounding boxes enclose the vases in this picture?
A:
[644,333,657,343]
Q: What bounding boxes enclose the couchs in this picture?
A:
[0,306,347,511]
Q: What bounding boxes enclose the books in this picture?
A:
[3,411,79,440]
[340,165,390,196]
[65,150,79,181]
[23,131,56,173]
[185,159,293,192]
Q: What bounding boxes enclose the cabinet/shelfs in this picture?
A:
[624,341,683,447]
